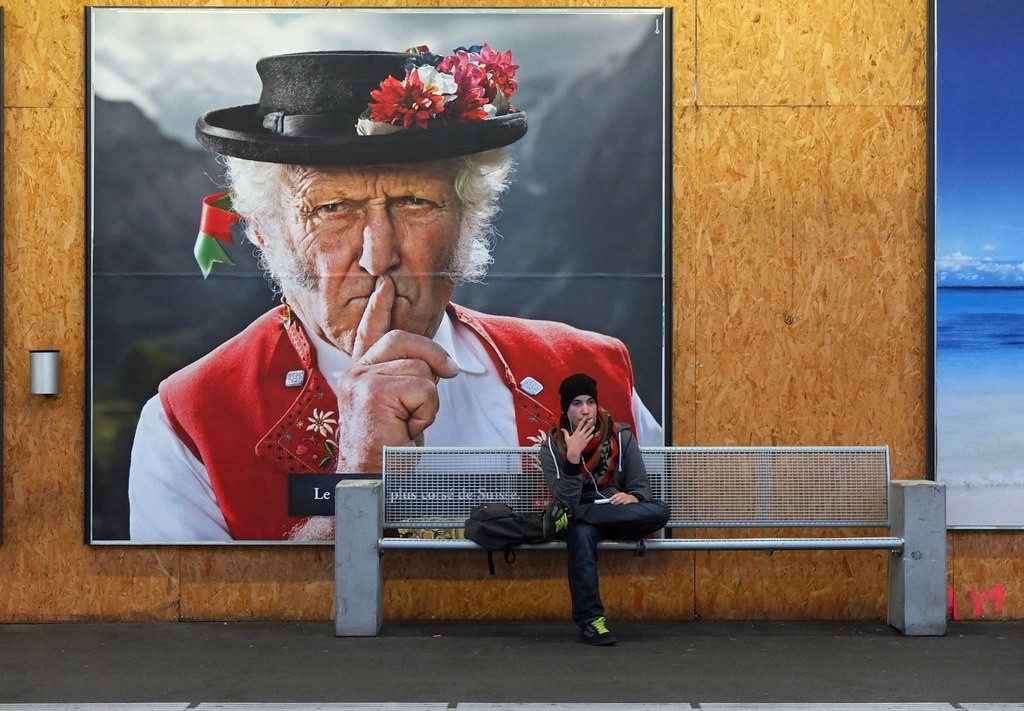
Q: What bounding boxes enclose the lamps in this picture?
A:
[29,350,60,397]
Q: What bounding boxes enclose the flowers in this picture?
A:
[352,41,520,137]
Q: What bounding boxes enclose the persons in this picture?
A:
[129,47,661,544]
[540,374,670,646]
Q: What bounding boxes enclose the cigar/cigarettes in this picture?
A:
[586,420,589,422]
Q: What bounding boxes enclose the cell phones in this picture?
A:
[594,498,611,504]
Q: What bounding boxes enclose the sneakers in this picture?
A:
[543,501,574,541]
[581,616,618,646]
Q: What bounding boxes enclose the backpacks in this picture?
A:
[463,503,543,576]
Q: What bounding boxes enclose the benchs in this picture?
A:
[334,444,948,636]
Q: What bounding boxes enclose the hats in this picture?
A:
[195,50,528,165]
[559,373,598,412]
